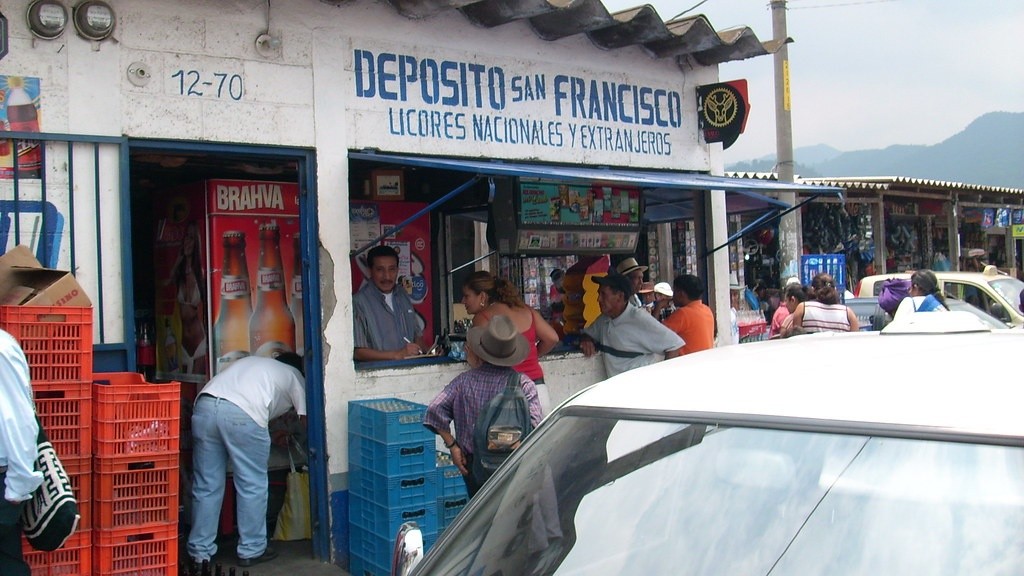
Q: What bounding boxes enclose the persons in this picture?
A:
[461,271,562,558]
[185,351,307,568]
[729,273,747,343]
[662,275,716,354]
[894,269,950,320]
[0,328,43,576]
[617,258,675,321]
[579,273,686,379]
[423,315,544,576]
[769,273,859,340]
[354,245,444,363]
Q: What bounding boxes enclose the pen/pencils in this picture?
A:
[403,336,424,356]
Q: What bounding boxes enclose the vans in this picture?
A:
[853,266,1024,328]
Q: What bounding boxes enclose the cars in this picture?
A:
[402,311,1024,576]
[843,297,1015,331]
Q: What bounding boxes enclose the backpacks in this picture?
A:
[471,372,532,482]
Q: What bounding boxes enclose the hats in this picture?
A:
[466,314,530,367]
[591,273,634,294]
[638,282,654,294]
[786,276,801,287]
[654,282,674,297]
[616,258,649,275]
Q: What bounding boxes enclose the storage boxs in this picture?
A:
[436,495,471,528]
[30,381,93,460]
[0,305,93,382]
[347,397,437,445]
[347,521,469,576]
[0,245,92,348]
[347,432,436,477]
[348,494,438,540]
[0,452,182,576]
[349,463,438,507]
[92,371,181,459]
[437,450,468,495]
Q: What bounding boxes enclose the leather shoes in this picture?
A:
[193,554,215,568]
[238,546,278,566]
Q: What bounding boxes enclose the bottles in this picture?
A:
[360,399,467,520]
[164,317,178,369]
[181,306,209,407]
[249,223,297,360]
[214,231,254,375]
[178,555,250,576]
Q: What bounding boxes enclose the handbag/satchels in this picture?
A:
[20,416,80,553]
[271,435,312,540]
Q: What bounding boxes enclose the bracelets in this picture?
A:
[443,439,456,448]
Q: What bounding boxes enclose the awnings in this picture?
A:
[351,151,845,258]
[727,191,793,240]
